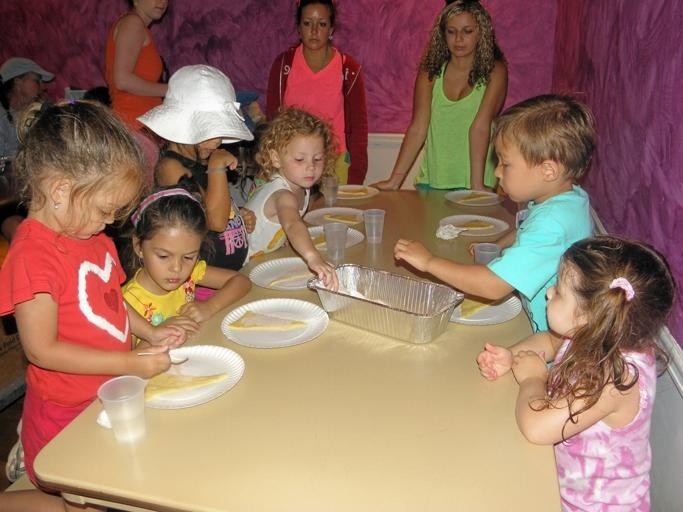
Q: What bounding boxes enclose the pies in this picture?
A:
[310,233,328,251]
[459,292,505,323]
[338,188,370,197]
[227,310,309,333]
[323,211,364,226]
[457,192,495,204]
[453,220,495,231]
[143,372,232,403]
[269,265,317,285]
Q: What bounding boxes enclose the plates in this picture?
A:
[221,298,330,348]
[249,257,317,290]
[146,346,245,411]
[303,207,364,225]
[439,215,510,237]
[336,185,379,199]
[307,226,365,251]
[445,190,504,207]
[449,295,522,326]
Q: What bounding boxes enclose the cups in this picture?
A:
[474,243,500,265]
[323,177,337,205]
[97,376,146,443]
[326,224,347,260]
[364,209,386,243]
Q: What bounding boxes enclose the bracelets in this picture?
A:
[205,168,228,173]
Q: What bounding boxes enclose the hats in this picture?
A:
[1,56,57,84]
[134,65,255,146]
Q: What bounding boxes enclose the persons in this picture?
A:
[476,237,676,512]
[246,106,338,288]
[369,1,508,192]
[0,58,54,168]
[116,186,253,348]
[1,99,185,512]
[137,64,256,273]
[393,93,600,371]
[108,0,170,139]
[267,0,368,186]
